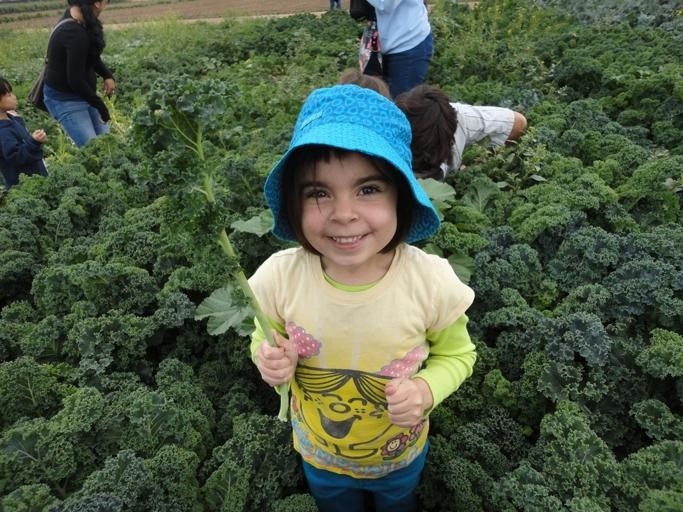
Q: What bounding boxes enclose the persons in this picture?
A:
[339,68,390,99]
[0,77,50,191]
[28,0,115,149]
[349,0,434,98]
[394,84,528,182]
[248,85,477,512]
[329,0,341,9]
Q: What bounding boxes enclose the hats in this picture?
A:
[264,85,441,247]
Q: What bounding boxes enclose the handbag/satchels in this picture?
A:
[28,60,48,112]
[358,21,384,76]
